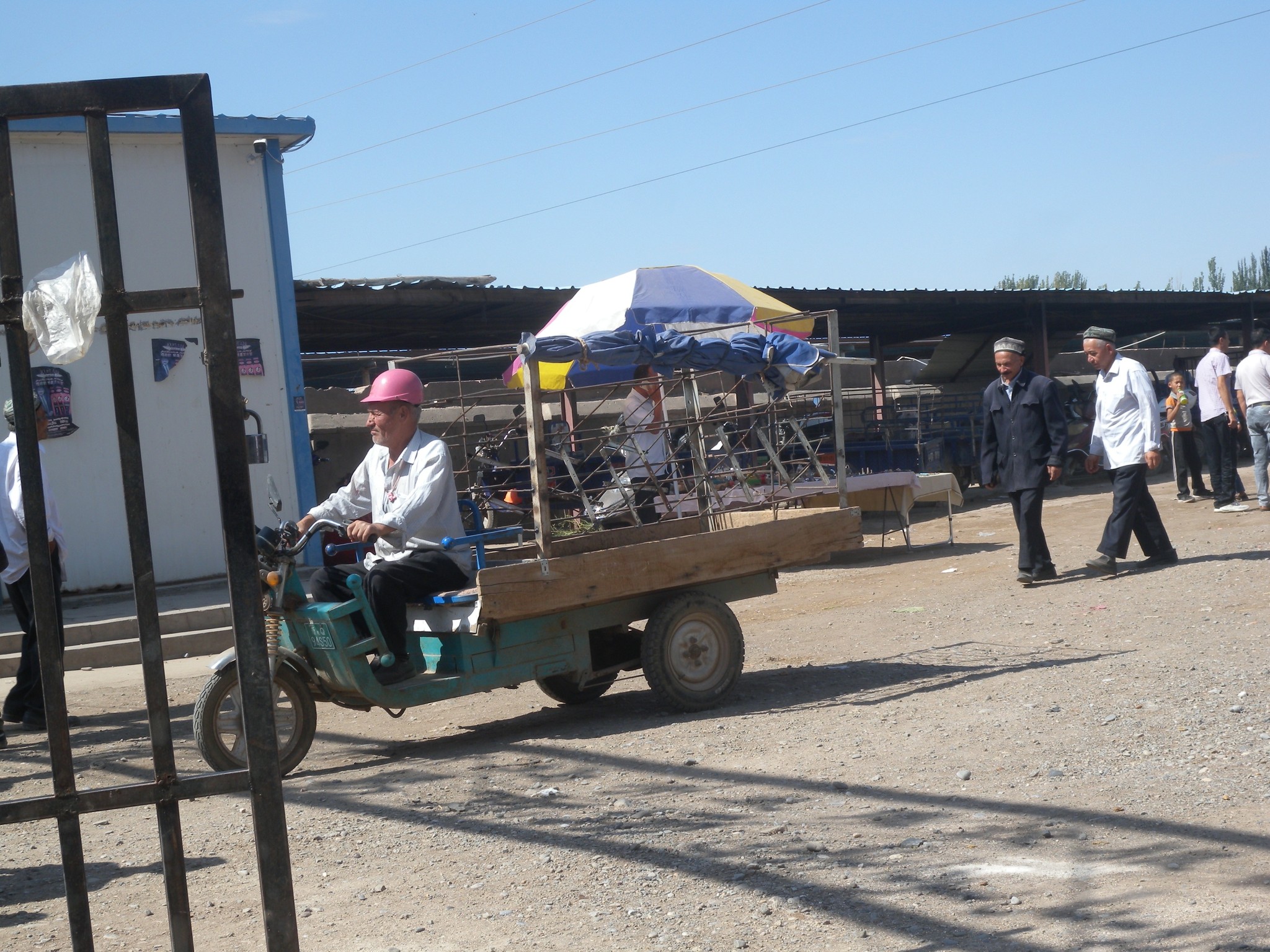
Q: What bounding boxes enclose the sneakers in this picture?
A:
[1192,488,1215,501]
[1177,495,1195,503]
[1214,500,1250,512]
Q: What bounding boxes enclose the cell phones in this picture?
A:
[639,380,650,391]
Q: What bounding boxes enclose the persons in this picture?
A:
[623,363,668,526]
[0,392,83,734]
[1233,404,1250,502]
[1083,325,1180,575]
[1234,327,1270,511]
[273,368,474,686]
[1166,372,1213,502]
[980,336,1069,582]
[1194,326,1249,513]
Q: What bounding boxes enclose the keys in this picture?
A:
[1225,411,1229,421]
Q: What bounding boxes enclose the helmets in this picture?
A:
[360,368,424,409]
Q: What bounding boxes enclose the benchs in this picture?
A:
[323,499,524,610]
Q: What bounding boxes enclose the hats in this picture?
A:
[4,392,41,426]
[992,336,1026,357]
[1082,326,1115,344]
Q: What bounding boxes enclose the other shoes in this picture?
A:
[0,703,81,731]
[1032,566,1058,581]
[1260,506,1270,511]
[369,652,416,687]
[1016,567,1034,583]
[1234,492,1249,502]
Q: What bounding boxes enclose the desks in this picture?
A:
[802,470,965,554]
[647,467,917,556]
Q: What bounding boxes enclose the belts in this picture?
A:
[1246,401,1270,408]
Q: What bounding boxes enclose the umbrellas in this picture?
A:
[501,265,817,392]
[517,324,878,403]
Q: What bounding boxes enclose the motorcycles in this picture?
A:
[456,367,1254,555]
[191,309,869,781]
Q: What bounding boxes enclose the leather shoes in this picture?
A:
[1086,556,1118,576]
[1137,548,1178,569]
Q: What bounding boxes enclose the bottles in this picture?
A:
[797,464,804,483]
